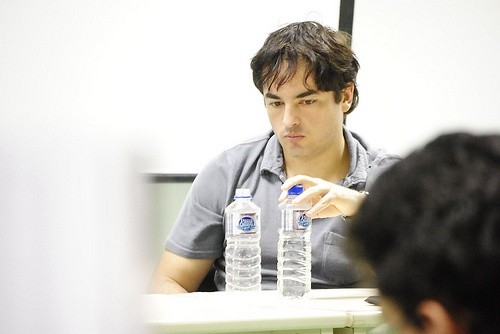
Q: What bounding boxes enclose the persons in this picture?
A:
[347,132,500,334]
[154,21,405,294]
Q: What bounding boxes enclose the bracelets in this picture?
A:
[341,191,369,224]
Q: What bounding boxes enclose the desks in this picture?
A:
[144,288,382,334]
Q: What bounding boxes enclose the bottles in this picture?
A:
[223,188,262,300]
[277,184,312,300]
